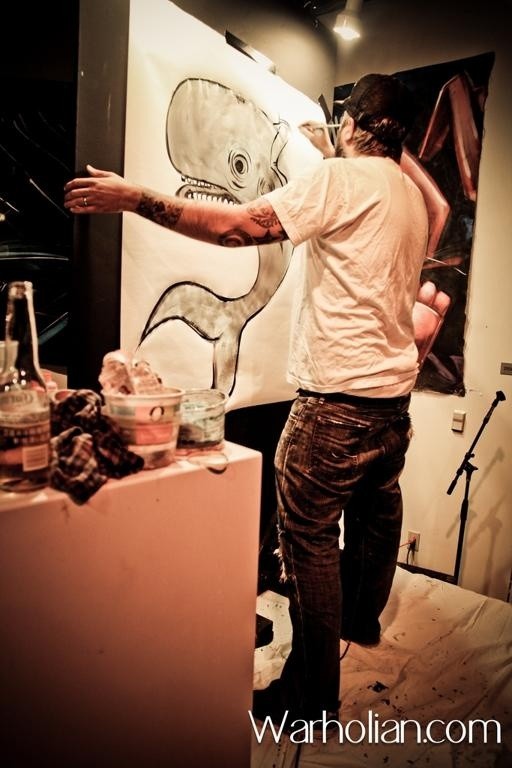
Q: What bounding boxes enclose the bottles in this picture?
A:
[0,279,57,498]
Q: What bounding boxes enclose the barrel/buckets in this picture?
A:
[180,391,228,447]
[101,388,186,468]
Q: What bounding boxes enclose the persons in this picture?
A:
[64,72,430,738]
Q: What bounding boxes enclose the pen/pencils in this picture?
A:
[298,124,342,128]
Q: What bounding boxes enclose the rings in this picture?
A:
[84,196,88,206]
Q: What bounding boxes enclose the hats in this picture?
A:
[331,72,419,145]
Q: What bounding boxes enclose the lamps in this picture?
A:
[333,0,363,42]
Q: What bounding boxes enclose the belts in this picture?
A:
[296,386,408,408]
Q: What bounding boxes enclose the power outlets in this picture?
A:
[407,530,420,551]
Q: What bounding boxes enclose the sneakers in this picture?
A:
[252,624,382,736]
